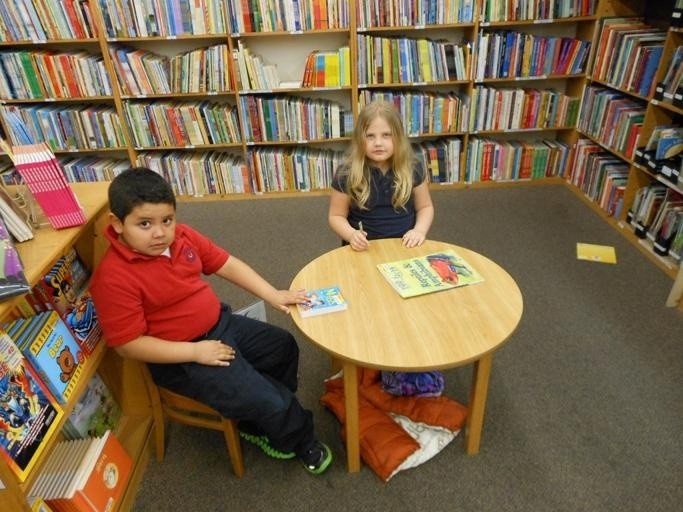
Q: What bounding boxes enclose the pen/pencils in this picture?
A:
[358,220,363,232]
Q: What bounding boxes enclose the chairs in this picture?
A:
[125,329,246,477]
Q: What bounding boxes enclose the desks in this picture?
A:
[289,238,524,476]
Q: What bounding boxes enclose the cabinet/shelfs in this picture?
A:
[566,0,683,307]
[0,1,566,201]
[0,180,168,511]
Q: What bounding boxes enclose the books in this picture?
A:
[569,0,682,197]
[576,241,618,266]
[377,248,486,300]
[131,0,355,197]
[356,0,599,185]
[590,198,682,265]
[1,199,133,512]
[293,285,347,318]
[1,0,132,198]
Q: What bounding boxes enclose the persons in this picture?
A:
[325,100,435,251]
[89,167,333,475]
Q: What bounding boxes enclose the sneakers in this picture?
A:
[240,428,296,459]
[300,439,333,475]
